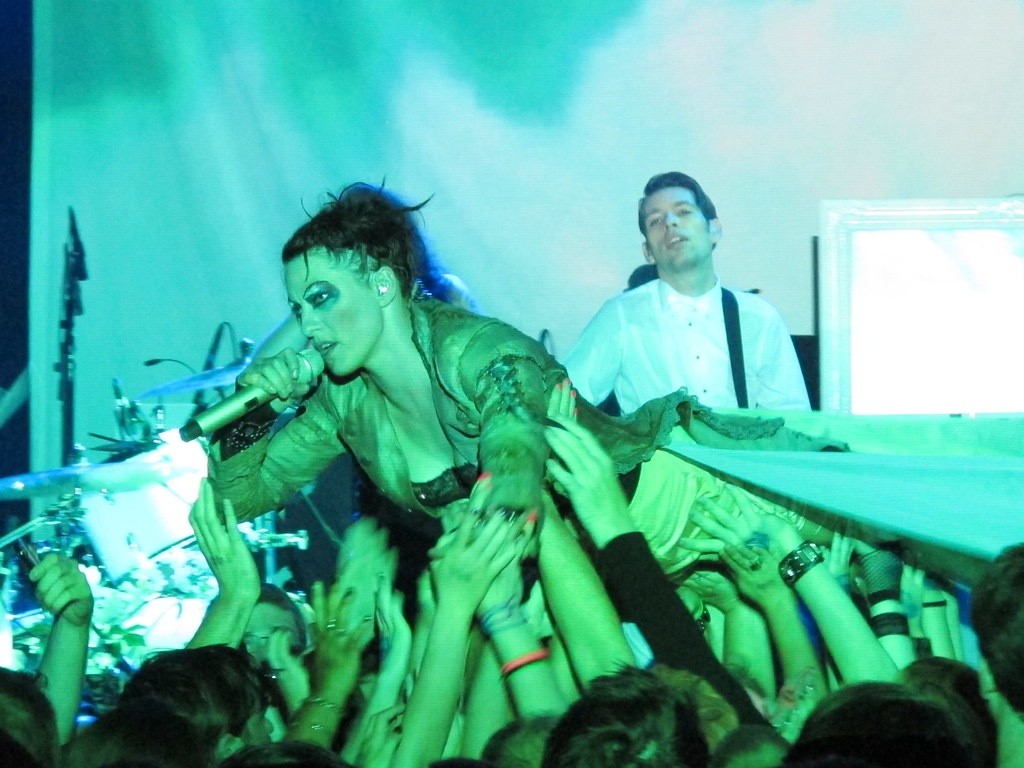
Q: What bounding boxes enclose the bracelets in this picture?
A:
[503,648,548,674]
[477,601,529,633]
[306,696,343,713]
[696,605,711,633]
[923,602,946,606]
[777,539,824,587]
[868,613,909,636]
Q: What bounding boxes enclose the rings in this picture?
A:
[749,556,762,570]
[325,619,337,629]
[695,577,701,583]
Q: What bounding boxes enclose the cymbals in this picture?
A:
[132,357,253,403]
[0,461,181,500]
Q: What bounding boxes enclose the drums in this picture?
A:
[1,536,97,647]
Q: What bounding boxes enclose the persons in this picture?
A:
[206,182,639,581]
[0,421,1024,768]
[563,174,814,420]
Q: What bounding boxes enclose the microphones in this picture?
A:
[70,208,87,281]
[179,348,325,442]
[192,326,224,405]
